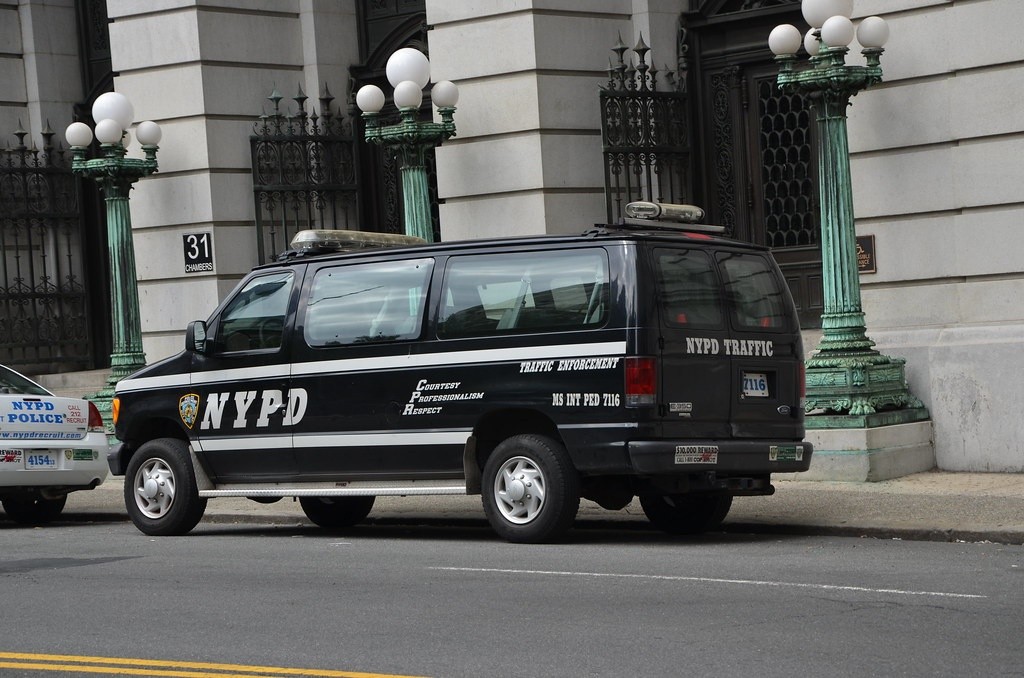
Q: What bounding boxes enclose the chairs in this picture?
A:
[304,265,775,345]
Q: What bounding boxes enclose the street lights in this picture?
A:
[67,92,162,442]
[765,0,927,427]
[356,46,460,235]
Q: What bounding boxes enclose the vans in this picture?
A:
[107,201,813,543]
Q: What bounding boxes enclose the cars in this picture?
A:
[0,365,108,520]
